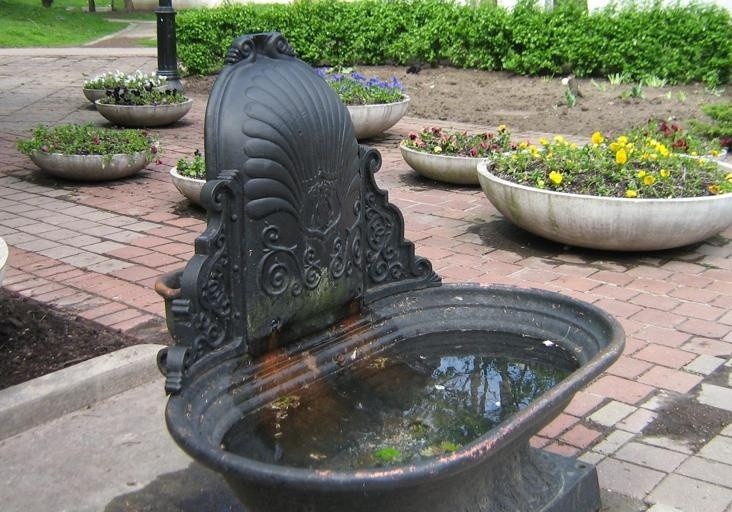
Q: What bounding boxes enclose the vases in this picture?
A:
[169,162,206,207]
[478,151,731,252]
